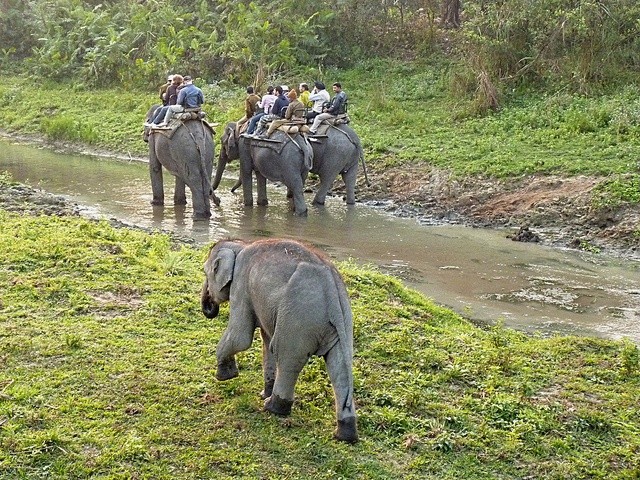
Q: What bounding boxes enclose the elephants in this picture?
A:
[286,121,374,206]
[210,121,316,217]
[200,235,362,445]
[143,119,222,221]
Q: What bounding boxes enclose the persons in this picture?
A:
[145,74,204,126]
[235,80,348,140]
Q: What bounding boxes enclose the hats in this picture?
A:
[288,90,298,99]
[313,81,325,89]
[167,75,174,81]
